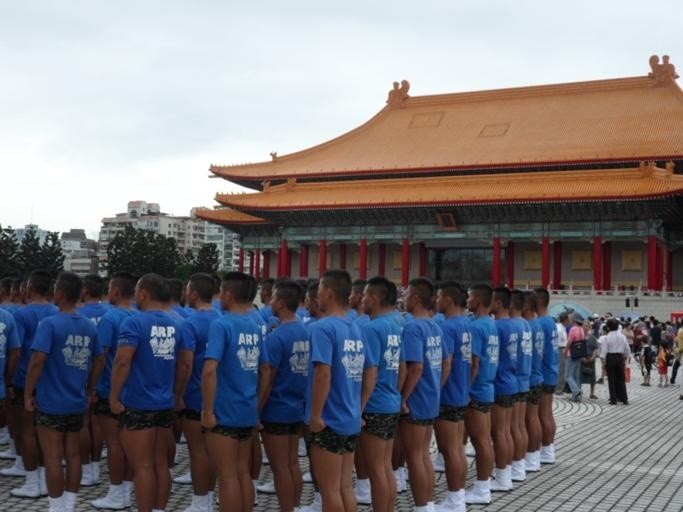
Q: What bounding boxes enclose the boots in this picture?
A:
[91,484,125,511]
[122,480,135,508]
[172,439,555,512]
[90,461,102,485]
[0,427,10,446]
[0,455,25,478]
[9,470,40,499]
[0,441,16,459]
[37,466,48,497]
[79,464,93,486]
